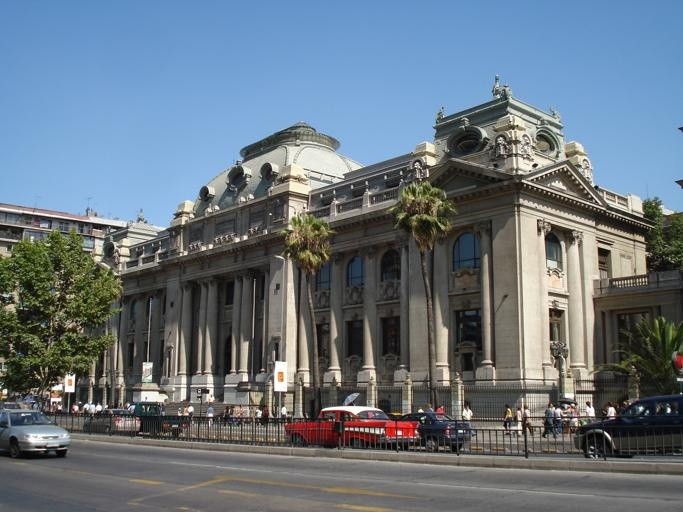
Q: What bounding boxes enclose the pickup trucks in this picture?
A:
[126,400,192,439]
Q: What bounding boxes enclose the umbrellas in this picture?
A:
[342,392,363,406]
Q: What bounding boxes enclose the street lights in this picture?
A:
[273,252,289,423]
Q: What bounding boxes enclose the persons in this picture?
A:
[178,400,291,431]
[513,407,524,436]
[523,404,536,434]
[418,404,445,423]
[30,394,168,416]
[460,403,478,435]
[503,404,514,436]
[542,402,633,435]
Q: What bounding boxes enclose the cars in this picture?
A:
[283,404,423,447]
[573,393,683,460]
[0,400,72,460]
[393,411,471,454]
[81,407,143,437]
